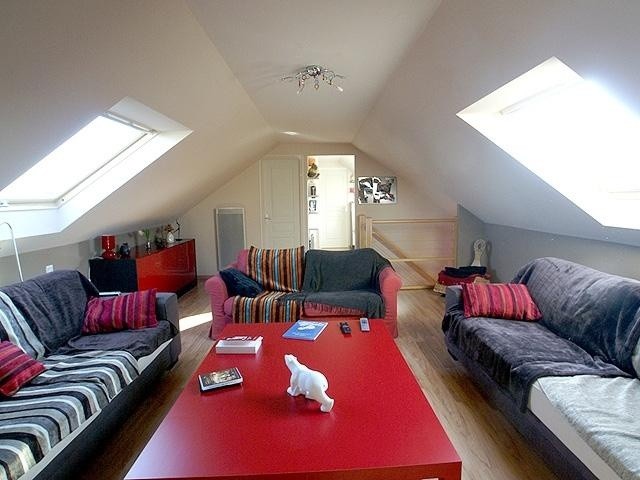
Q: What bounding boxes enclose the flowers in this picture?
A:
[162,223,174,233]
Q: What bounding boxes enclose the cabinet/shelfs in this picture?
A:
[88,238,199,301]
[307,176,320,231]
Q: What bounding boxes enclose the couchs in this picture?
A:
[443,257,640,480]
[204,247,402,341]
[0,269,182,480]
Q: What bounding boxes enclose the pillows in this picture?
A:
[461,280,543,321]
[0,338,48,398]
[219,266,263,298]
[80,287,159,336]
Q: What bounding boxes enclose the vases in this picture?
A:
[166,231,175,244]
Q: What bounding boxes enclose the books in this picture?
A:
[281,320,328,342]
[215,335,264,355]
[196,366,245,393]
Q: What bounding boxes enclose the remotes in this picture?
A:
[339,321,351,334]
[359,317,370,332]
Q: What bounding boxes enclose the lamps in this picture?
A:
[278,64,351,95]
[101,234,118,261]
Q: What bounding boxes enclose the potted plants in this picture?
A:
[142,227,152,249]
